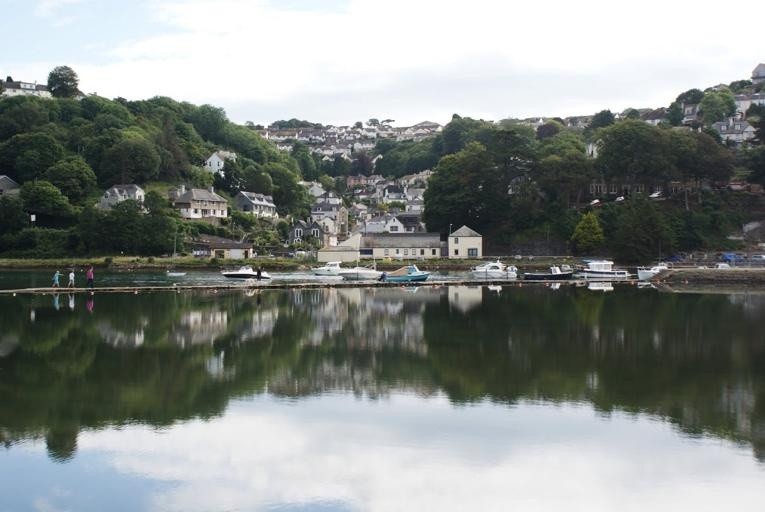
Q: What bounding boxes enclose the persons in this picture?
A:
[256,293,263,310]
[50,269,64,287]
[87,290,95,315]
[86,265,94,288]
[67,268,76,287]
[254,265,265,282]
[52,292,61,311]
[68,292,76,313]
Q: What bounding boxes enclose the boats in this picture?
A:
[384,262,431,282]
[167,270,188,277]
[699,261,733,269]
[635,262,673,282]
[471,258,517,279]
[310,260,350,278]
[220,265,271,279]
[337,258,386,280]
[523,264,573,280]
[559,258,633,279]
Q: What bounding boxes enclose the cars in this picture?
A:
[669,254,686,262]
[722,253,744,262]
[751,253,765,262]
